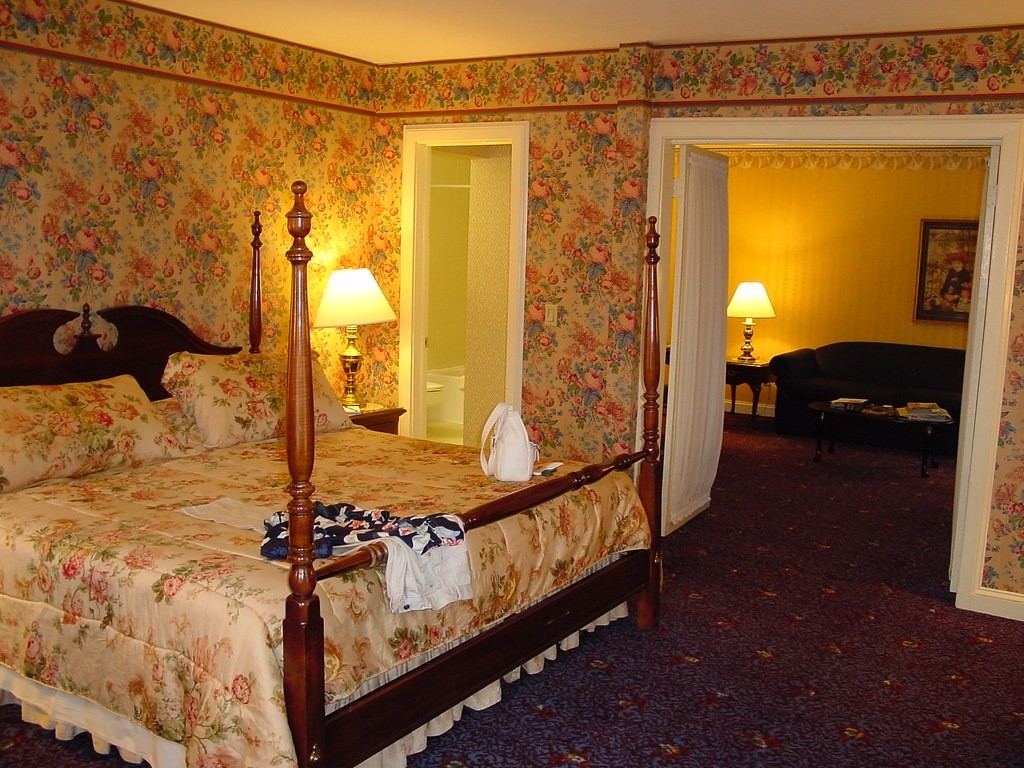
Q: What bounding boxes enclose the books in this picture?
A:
[908,403,939,412]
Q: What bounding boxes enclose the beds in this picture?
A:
[0,181,668,766]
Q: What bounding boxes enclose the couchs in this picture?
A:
[768,341,966,456]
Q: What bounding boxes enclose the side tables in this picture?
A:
[725,360,768,424]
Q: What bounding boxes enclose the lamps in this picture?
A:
[726,281,776,360]
[311,268,398,407]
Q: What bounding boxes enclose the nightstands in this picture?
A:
[343,407,408,434]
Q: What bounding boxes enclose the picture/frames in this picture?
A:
[913,219,980,324]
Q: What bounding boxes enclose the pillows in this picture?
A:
[161,346,354,450]
[0,374,188,492]
[152,398,204,456]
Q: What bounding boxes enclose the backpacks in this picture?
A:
[480,403,539,483]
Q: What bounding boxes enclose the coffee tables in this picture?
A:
[810,402,956,478]
